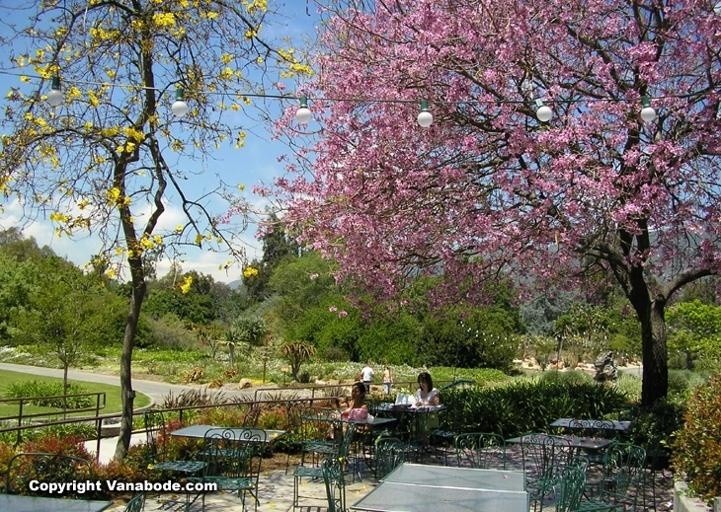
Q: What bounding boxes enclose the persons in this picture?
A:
[360,362,376,394]
[409,372,442,407]
[336,381,369,424]
[380,366,394,395]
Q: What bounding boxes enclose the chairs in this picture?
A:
[0,391,655,512]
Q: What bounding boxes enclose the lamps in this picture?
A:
[640,96,656,122]
[296,96,311,123]
[172,89,188,117]
[536,99,552,122]
[418,100,434,128]
[47,76,62,105]
